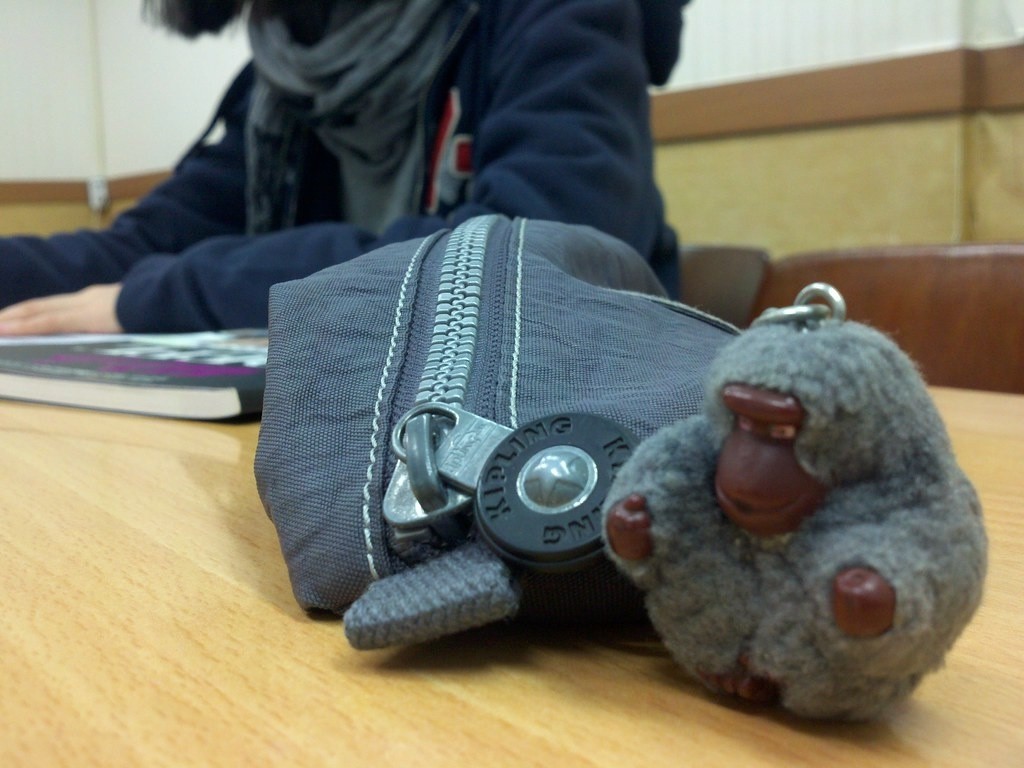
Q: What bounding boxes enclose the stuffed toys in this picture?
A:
[599,315,990,724]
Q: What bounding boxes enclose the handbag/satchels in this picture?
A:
[252,213,743,651]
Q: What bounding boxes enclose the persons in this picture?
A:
[0,0,699,334]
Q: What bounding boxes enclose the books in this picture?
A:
[0,327,271,426]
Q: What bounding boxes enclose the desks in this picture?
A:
[2,378,1023,768]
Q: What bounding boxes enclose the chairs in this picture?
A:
[669,248,1024,395]
